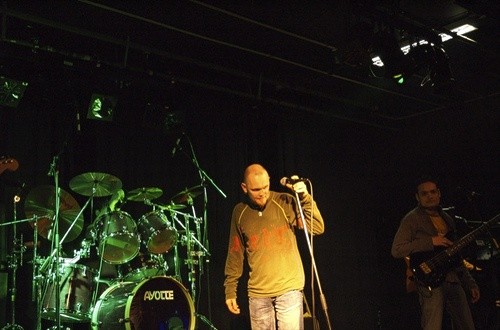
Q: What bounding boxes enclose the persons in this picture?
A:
[391,177,480,330]
[223,164,324,330]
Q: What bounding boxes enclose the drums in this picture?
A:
[91,275,196,330]
[94,210,141,265]
[136,211,179,255]
[40,264,100,324]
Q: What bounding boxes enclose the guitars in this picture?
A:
[409,214,500,286]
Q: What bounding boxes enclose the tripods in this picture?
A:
[1,203,24,330]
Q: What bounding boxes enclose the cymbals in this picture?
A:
[124,183,165,202]
[167,184,213,204]
[160,205,189,211]
[67,172,124,198]
[23,184,83,244]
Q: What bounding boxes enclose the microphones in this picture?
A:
[172,132,186,155]
[279,177,309,186]
[47,151,63,175]
